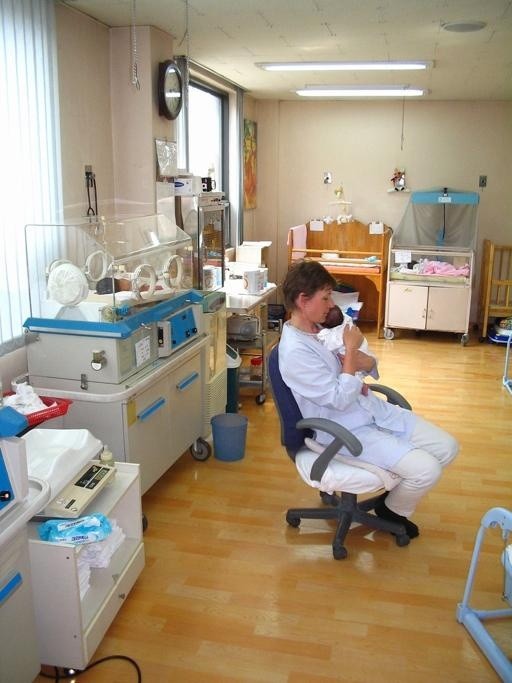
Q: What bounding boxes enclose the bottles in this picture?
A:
[101,444,115,484]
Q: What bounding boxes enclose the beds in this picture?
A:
[474,239,512,343]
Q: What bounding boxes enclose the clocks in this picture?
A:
[159,60,183,120]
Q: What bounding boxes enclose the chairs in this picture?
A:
[266,344,413,560]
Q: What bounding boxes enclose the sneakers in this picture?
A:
[376,505,418,537]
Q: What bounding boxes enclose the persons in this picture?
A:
[93,277,163,296]
[318,306,369,396]
[277,261,458,537]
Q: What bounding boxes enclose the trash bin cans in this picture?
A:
[226,344,242,413]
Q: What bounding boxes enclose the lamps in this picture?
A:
[289,85,431,96]
[255,61,435,71]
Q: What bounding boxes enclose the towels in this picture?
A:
[76,518,126,601]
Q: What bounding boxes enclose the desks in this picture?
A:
[215,279,282,404]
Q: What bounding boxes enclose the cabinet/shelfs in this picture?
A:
[286,219,393,339]
[383,236,475,347]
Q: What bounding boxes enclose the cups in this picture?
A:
[188,176,216,195]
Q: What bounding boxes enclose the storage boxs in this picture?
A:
[260,302,268,331]
[236,241,272,268]
[174,177,202,196]
